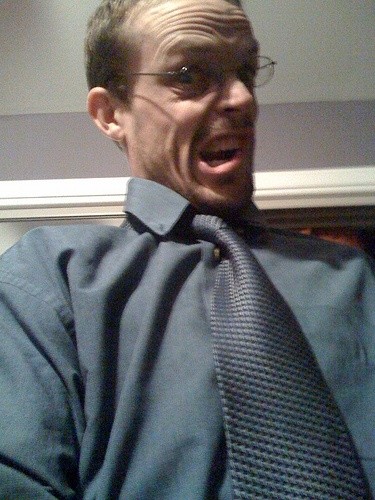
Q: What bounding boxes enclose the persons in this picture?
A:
[0,0,375,500]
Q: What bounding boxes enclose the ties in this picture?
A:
[189,210,373,499]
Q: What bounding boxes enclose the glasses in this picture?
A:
[108,56,277,94]
